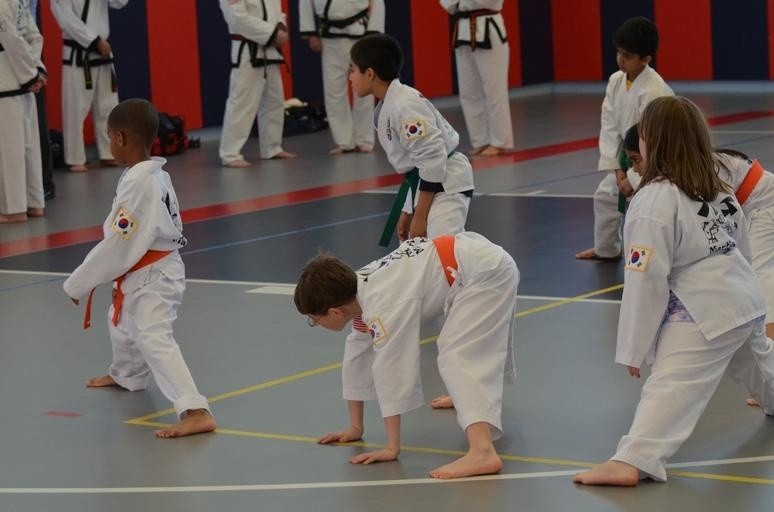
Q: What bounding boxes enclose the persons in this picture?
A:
[576,16,676,261]
[348,32,475,245]
[51,0,130,172]
[0,0,49,224]
[625,123,774,406]
[438,0,514,156]
[573,96,774,487]
[298,0,387,153]
[294,230,522,481]
[61,98,218,439]
[218,0,295,168]
[27,2,58,201]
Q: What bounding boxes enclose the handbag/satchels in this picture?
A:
[271,97,327,136]
[150,112,188,157]
